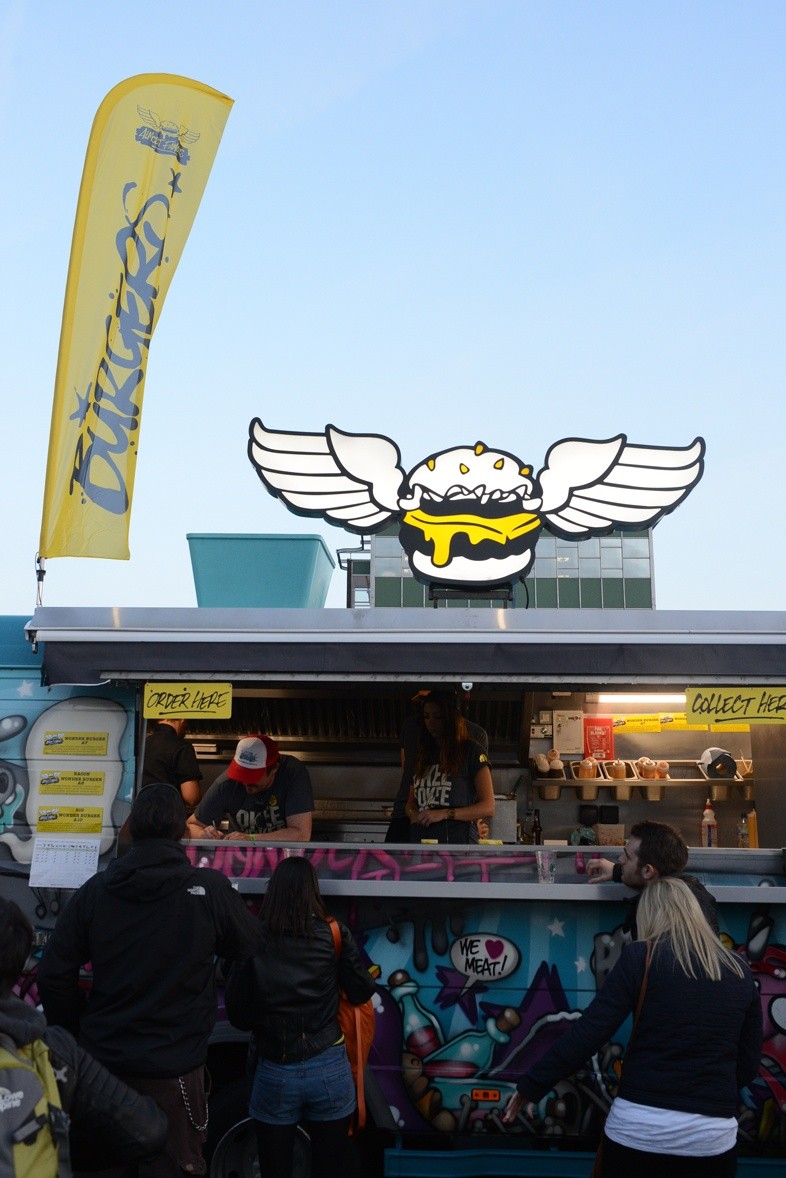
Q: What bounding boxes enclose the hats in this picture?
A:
[227,732,281,783]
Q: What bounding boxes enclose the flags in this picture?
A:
[38,73,236,560]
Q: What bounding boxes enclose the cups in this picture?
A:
[536,850,556,884]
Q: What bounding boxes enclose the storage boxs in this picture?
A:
[592,824,625,846]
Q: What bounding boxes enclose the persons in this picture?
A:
[142,719,203,802]
[587,820,720,942]
[185,734,314,841]
[505,877,764,1178]
[224,857,375,1178]
[37,785,260,1178]
[0,896,171,1178]
[400,690,495,844]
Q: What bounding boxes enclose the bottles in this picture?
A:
[579,755,597,779]
[533,750,563,779]
[737,807,760,847]
[532,807,544,845]
[638,757,670,779]
[702,798,718,847]
[613,757,626,779]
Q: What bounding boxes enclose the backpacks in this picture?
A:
[325,918,374,1135]
[0,1035,70,1178]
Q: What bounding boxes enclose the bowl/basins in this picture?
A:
[734,759,754,777]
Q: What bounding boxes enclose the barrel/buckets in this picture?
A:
[488,799,517,842]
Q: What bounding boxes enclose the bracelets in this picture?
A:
[448,809,455,820]
[249,834,255,840]
